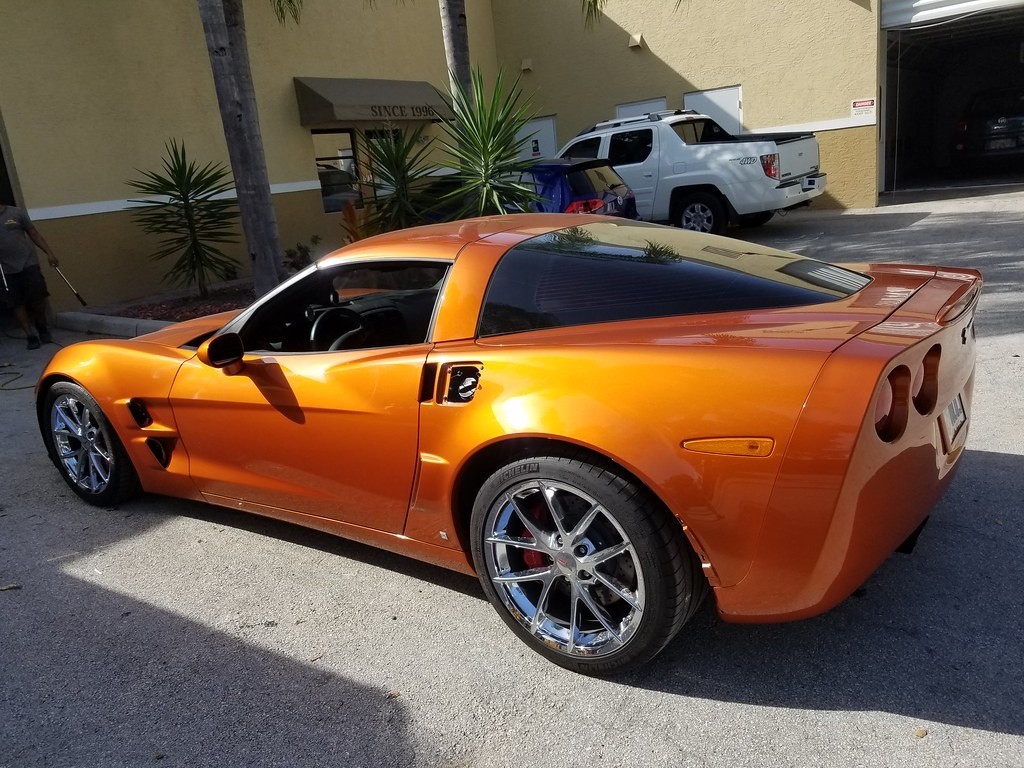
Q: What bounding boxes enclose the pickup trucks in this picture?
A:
[551,108,828,235]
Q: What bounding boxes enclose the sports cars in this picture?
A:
[34,209,983,679]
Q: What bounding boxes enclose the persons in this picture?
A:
[543,259,603,327]
[0,203,60,351]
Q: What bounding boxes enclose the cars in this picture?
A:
[956,93,1024,160]
[317,163,396,208]
[425,155,643,223]
